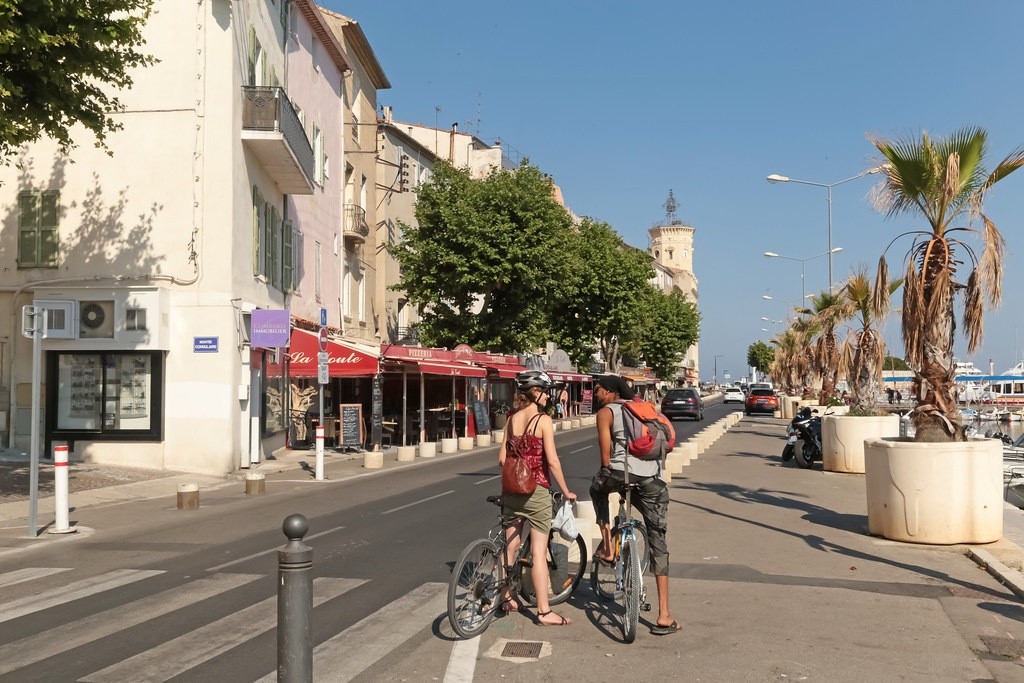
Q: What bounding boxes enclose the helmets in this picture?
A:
[513,371,557,390]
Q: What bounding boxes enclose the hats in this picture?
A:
[598,374,637,400]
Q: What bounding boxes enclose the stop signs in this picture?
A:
[318,327,329,353]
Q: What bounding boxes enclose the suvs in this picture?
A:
[660,387,705,422]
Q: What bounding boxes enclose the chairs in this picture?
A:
[686,392,692,396]
[363,413,452,448]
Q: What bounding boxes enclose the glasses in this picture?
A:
[537,389,550,395]
[592,386,604,393]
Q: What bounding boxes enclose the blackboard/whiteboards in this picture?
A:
[340,404,362,444]
[473,400,486,432]
[479,402,491,429]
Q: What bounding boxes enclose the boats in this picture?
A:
[897,376,1024,489]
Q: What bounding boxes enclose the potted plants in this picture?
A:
[490,400,510,430]
[863,124,1024,543]
[780,262,903,473]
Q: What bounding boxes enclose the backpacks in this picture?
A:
[501,412,545,495]
[601,397,680,483]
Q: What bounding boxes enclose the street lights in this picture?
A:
[765,161,893,304]
[712,355,724,386]
[761,293,816,398]
[763,246,844,385]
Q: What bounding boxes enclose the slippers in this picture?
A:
[651,621,681,634]
[592,551,616,568]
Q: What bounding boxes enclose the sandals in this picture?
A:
[501,597,528,613]
[535,610,572,626]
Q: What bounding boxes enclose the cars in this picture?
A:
[722,387,747,404]
[734,380,780,416]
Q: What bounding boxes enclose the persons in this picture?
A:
[588,376,683,636]
[494,369,577,627]
[897,391,902,403]
[887,388,893,404]
[837,390,850,400]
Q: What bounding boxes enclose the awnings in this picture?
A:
[544,371,593,382]
[262,327,488,447]
[476,361,530,378]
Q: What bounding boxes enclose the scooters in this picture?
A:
[781,402,836,469]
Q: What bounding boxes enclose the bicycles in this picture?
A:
[590,483,653,643]
[447,487,588,639]
